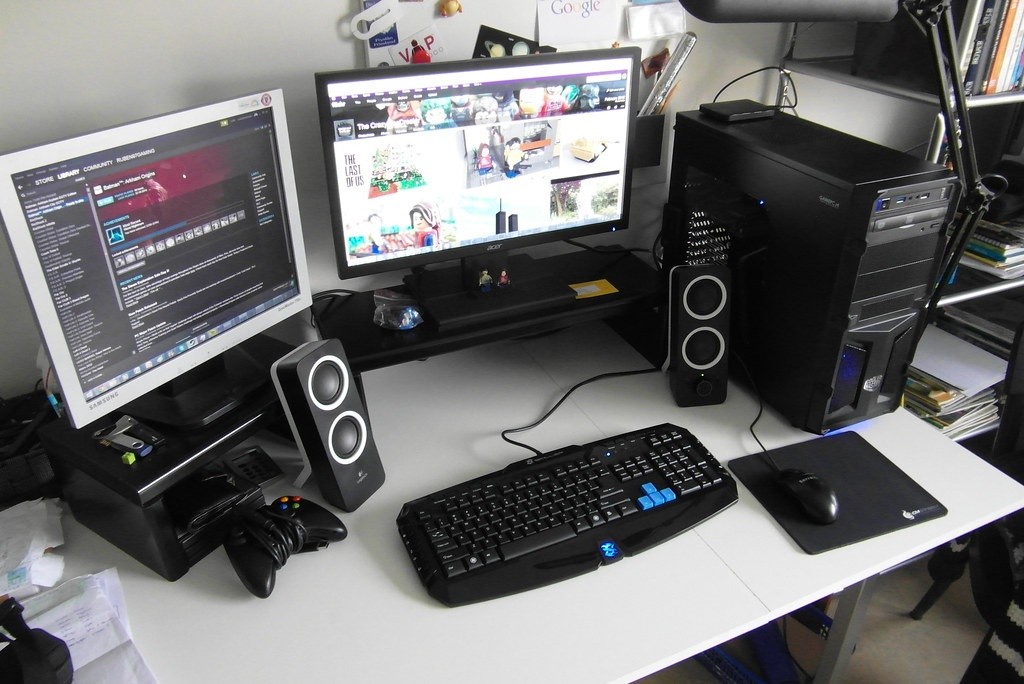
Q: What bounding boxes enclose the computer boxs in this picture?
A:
[659,108,966,436]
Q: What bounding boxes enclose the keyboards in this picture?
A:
[395,424,739,608]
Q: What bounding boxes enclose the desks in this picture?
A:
[36,323,1024,684]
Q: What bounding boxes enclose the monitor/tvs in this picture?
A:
[0,86,313,428]
[312,46,641,336]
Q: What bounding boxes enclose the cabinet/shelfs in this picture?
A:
[776,0,1024,443]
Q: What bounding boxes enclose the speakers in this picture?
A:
[271,339,384,511]
[662,264,730,408]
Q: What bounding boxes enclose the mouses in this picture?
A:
[768,468,841,524]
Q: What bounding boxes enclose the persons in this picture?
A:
[476,265,512,298]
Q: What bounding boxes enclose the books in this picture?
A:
[816,0,1024,445]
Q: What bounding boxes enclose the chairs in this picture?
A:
[910,327,1024,633]
